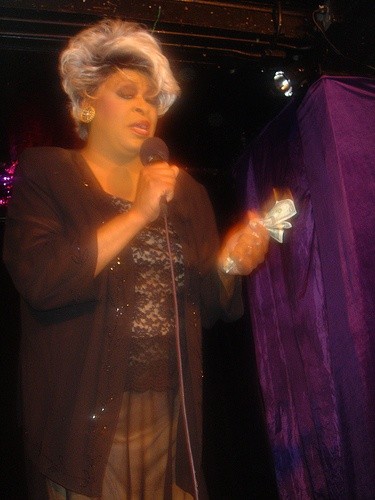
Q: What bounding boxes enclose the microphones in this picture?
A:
[139,137,170,213]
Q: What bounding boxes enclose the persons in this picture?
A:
[3,20,295,500]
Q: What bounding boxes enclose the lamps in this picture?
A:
[271,50,310,97]
